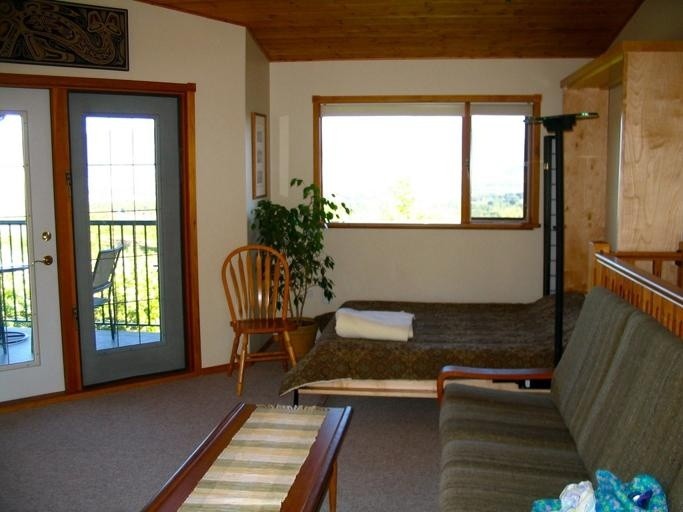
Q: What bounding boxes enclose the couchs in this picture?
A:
[438,284,682,511]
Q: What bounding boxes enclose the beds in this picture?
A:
[293,289,590,408]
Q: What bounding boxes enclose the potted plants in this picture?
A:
[251,177,351,367]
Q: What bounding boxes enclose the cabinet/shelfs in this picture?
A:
[560,38,682,301]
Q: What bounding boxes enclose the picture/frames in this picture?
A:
[251,113,268,200]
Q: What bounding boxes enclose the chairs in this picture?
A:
[221,244,298,398]
[90,246,123,342]
